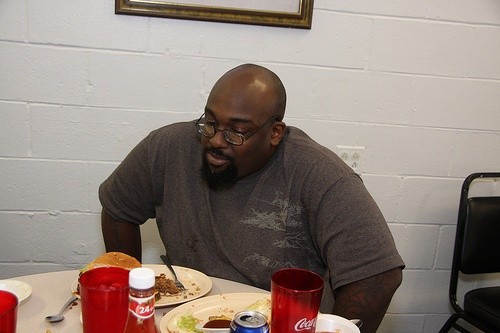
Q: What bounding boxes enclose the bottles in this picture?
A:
[124,267,159,333]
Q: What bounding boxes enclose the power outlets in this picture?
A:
[338,145,367,175]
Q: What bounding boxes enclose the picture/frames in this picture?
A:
[114,0,315,30]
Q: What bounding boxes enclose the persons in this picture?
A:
[99,65,406,333]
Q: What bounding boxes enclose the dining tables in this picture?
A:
[7,268,320,333]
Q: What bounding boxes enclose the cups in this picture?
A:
[78,267,129,333]
[0,290,18,333]
[271,268,326,333]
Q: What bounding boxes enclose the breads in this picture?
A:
[80,252,142,276]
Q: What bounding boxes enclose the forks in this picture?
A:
[160,254,189,290]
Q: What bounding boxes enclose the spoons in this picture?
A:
[45,295,78,322]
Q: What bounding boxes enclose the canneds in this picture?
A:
[229,310,270,333]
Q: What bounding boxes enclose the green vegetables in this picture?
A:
[178,314,204,333]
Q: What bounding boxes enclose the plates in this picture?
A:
[71,263,212,307]
[315,314,360,333]
[0,279,32,301]
[159,293,271,333]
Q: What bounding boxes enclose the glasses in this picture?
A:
[194,112,279,146]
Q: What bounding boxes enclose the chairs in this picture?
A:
[438,173,500,333]
